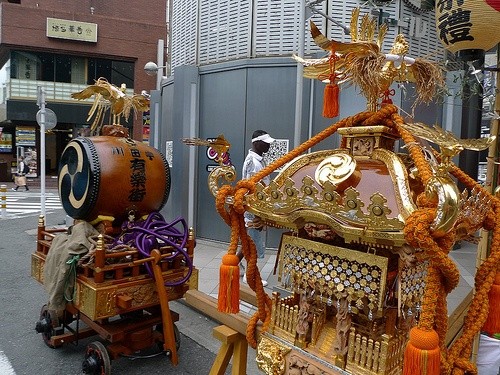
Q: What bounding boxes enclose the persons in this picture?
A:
[238,129,277,288]
[12,155,30,193]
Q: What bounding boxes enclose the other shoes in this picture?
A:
[243,273,267,287]
[236,252,245,275]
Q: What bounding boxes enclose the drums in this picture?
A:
[57,135,171,222]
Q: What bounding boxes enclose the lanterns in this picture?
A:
[435,0,500,60]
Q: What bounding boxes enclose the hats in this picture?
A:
[251,133,276,144]
